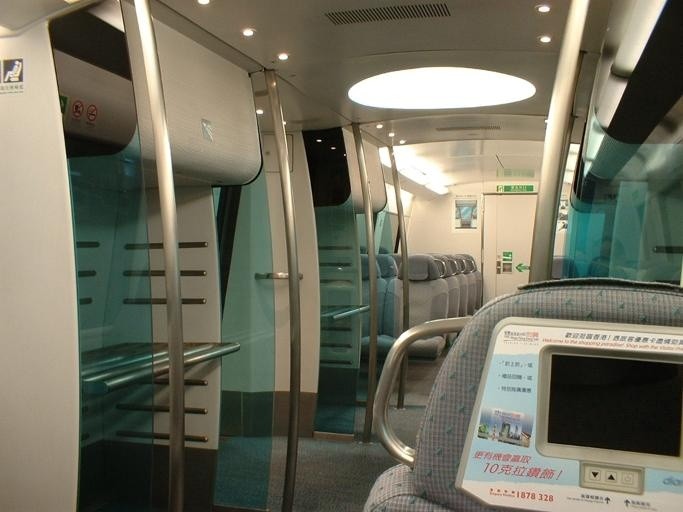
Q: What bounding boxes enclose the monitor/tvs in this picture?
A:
[537,344,683,474]
